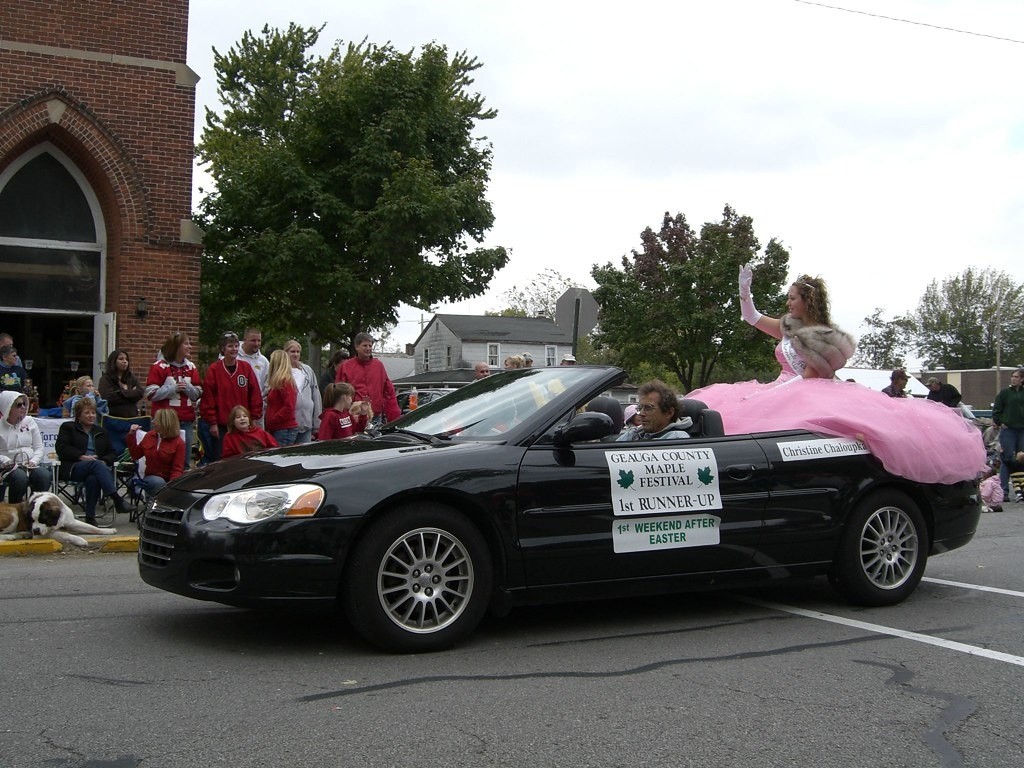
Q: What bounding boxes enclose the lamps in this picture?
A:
[136,296,147,322]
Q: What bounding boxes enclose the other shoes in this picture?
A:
[115,500,137,514]
[1003,494,1010,502]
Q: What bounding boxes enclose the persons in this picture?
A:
[98,350,145,418]
[221,327,270,430]
[738,263,856,379]
[616,379,693,442]
[548,354,588,413]
[55,397,136,526]
[199,330,263,462]
[925,376,962,407]
[980,466,1004,513]
[334,331,401,426]
[126,408,185,495]
[221,404,280,458]
[265,350,298,445]
[317,382,369,440]
[64,376,109,416]
[0,333,29,393]
[284,340,323,443]
[472,362,490,382]
[882,369,911,397]
[982,368,1024,502]
[504,352,533,369]
[145,331,203,471]
[0,390,51,504]
[319,351,348,395]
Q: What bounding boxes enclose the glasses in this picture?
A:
[174,333,180,339]
[8,354,17,357]
[224,333,237,338]
[902,378,908,383]
[635,404,666,415]
[14,402,25,408]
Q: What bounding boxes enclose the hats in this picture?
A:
[892,370,911,378]
[623,404,639,425]
[523,353,532,361]
[925,377,940,385]
[562,354,576,361]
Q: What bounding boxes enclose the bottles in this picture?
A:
[409,387,418,410]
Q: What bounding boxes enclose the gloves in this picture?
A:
[738,264,763,326]
[743,375,803,400]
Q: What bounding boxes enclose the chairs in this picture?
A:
[674,399,725,437]
[584,398,626,438]
[24,409,154,530]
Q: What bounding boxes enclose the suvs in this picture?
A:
[396,388,459,416]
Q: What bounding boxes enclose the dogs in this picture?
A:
[0,491,118,547]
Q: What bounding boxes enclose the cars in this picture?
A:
[958,409,995,433]
[138,366,982,655]
